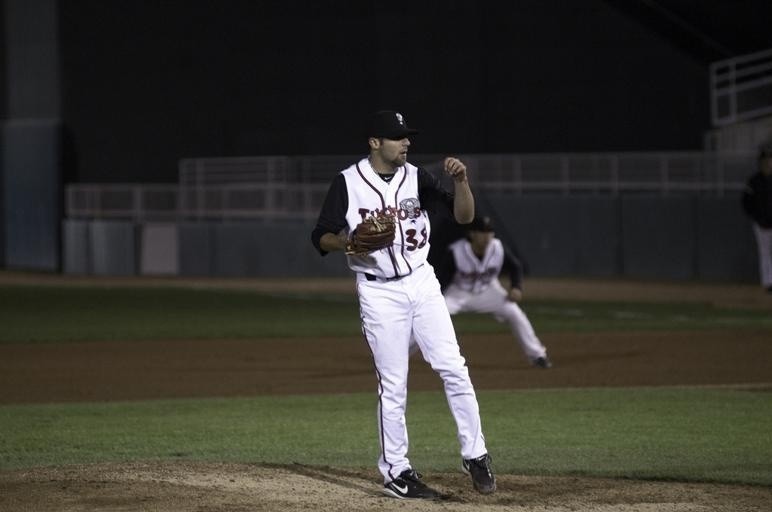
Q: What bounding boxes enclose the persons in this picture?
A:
[739,144,770,296]
[406,211,554,370]
[310,107,498,502]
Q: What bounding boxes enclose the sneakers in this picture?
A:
[536,356,552,368]
[384,468,447,501]
[462,454,496,494]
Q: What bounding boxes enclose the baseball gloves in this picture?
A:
[350,220,395,253]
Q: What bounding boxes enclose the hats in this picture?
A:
[372,111,419,140]
[474,217,495,231]
